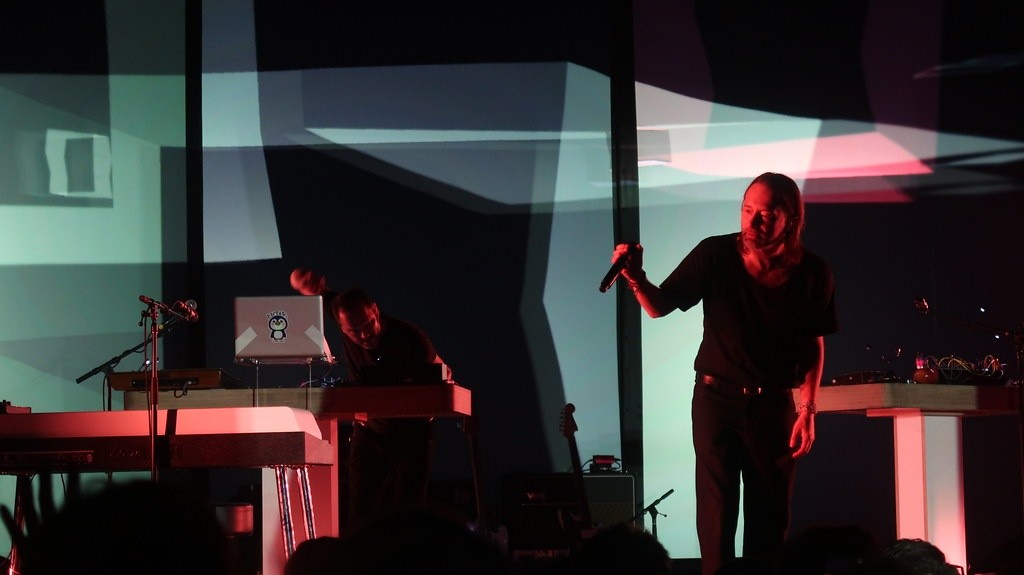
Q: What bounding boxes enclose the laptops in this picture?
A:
[235,295,328,358]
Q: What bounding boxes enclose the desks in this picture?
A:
[791,383,1024,575]
[126,387,475,575]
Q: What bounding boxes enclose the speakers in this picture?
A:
[492,469,644,560]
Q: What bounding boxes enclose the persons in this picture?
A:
[612,173,839,575]
[288,269,450,532]
[0,465,1024,575]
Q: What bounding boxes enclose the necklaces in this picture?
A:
[377,355,382,361]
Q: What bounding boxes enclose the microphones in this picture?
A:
[599,240,638,292]
[174,300,199,323]
[159,299,197,331]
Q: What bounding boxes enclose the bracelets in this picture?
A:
[628,271,645,295]
[798,401,817,413]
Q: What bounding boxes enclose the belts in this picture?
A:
[694,373,802,399]
